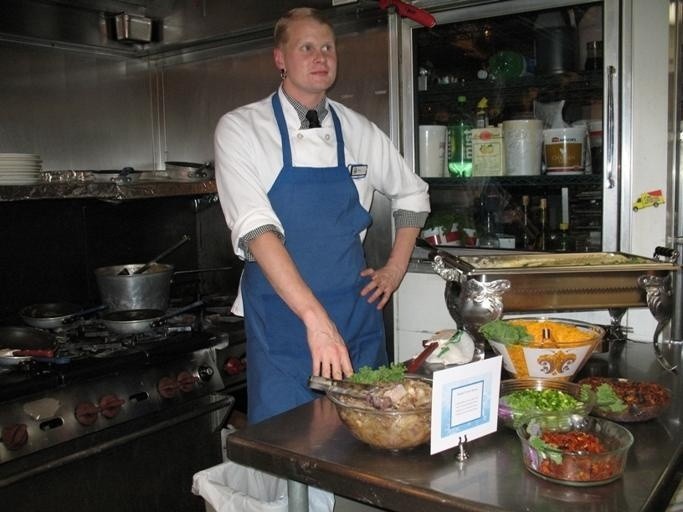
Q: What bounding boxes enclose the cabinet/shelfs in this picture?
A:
[397,0,633,274]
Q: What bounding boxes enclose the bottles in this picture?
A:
[478,211,501,250]
[514,195,576,253]
[477,68,497,81]
[447,94,476,177]
[472,98,492,128]
[584,40,604,70]
[490,50,537,77]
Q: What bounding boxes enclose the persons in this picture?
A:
[211,5,432,430]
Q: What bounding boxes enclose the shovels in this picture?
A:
[118,234,191,275]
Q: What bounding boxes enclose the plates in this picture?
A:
[1,151,45,186]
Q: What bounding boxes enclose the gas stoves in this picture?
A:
[0,295,247,488]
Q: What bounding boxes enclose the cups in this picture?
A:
[532,26,577,76]
[418,120,604,177]
[41,169,98,184]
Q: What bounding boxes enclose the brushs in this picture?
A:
[0,348,53,357]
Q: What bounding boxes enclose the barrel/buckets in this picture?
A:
[543,128,588,175]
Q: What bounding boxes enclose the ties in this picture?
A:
[305,109,322,128]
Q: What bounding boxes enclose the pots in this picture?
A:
[93,167,143,185]
[17,300,110,329]
[0,326,74,366]
[102,299,204,336]
[93,264,235,312]
[166,157,216,182]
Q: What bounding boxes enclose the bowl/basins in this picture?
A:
[516,414,634,486]
[576,377,674,424]
[412,345,486,373]
[483,315,608,389]
[496,375,596,430]
[327,375,434,455]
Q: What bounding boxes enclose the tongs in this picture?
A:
[435,247,474,272]
[306,374,385,400]
